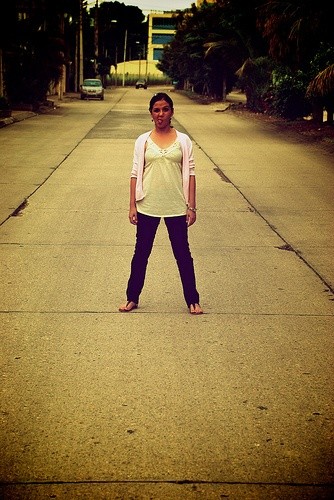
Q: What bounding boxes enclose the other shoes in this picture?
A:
[190,303,203,314]
[119,301,137,312]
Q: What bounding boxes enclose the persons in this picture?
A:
[118,92,203,314]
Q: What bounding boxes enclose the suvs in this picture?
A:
[79,78,104,100]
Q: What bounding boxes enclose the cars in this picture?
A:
[135,78,147,89]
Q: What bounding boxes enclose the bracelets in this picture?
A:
[186,203,198,211]
